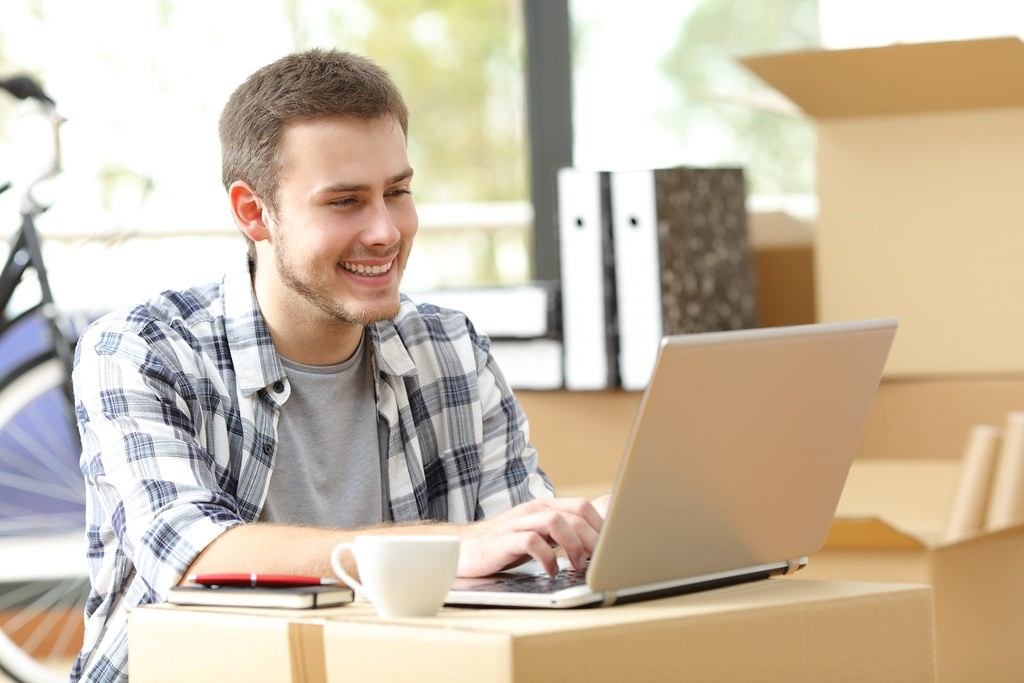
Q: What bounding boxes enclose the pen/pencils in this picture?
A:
[188,573,339,590]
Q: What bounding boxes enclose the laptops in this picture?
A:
[444,316,898,608]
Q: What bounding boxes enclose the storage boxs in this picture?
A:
[411,166,756,391]
[775,458,1024,683]
[731,36,1024,381]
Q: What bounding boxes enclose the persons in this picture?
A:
[65,46,610,682]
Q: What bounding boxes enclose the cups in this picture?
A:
[329,534,461,618]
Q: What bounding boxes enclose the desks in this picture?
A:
[122,579,935,683]
